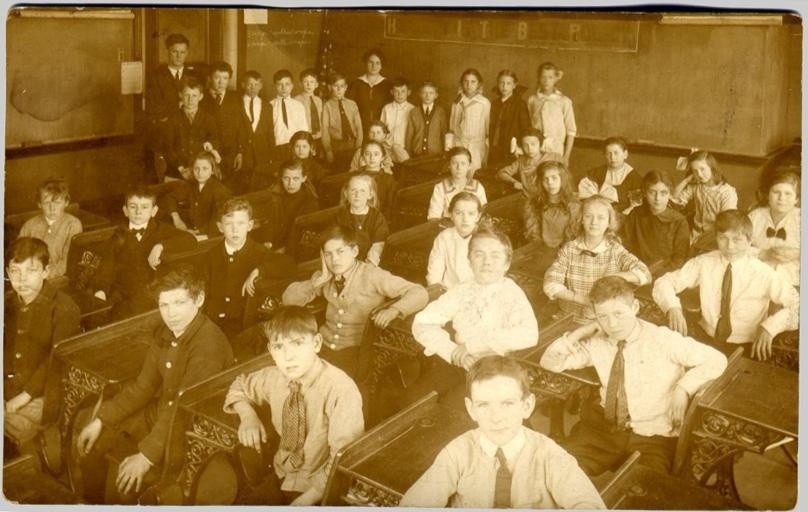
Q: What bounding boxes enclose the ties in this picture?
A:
[336,276,345,295]
[250,98,254,124]
[495,448,511,508]
[310,96,321,134]
[604,340,628,428]
[282,98,287,127]
[216,93,221,102]
[339,100,351,137]
[282,381,306,468]
[714,263,731,343]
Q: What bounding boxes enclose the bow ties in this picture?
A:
[767,227,786,239]
[132,228,144,235]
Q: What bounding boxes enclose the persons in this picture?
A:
[4,139,799,511]
[138,33,576,169]
